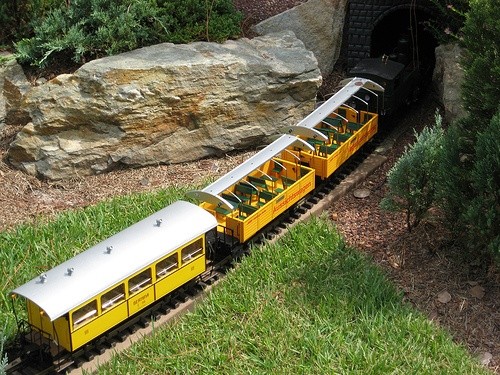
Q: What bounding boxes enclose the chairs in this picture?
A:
[60,86,377,326]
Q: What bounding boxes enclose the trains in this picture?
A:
[11,29,440,372]
